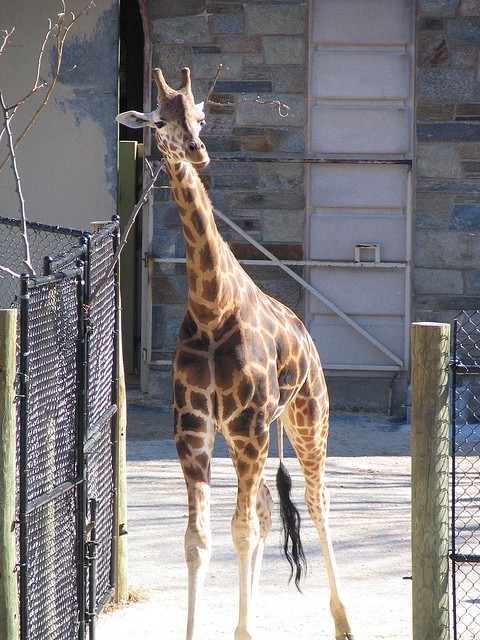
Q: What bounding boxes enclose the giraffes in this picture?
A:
[116,67,358,640]
